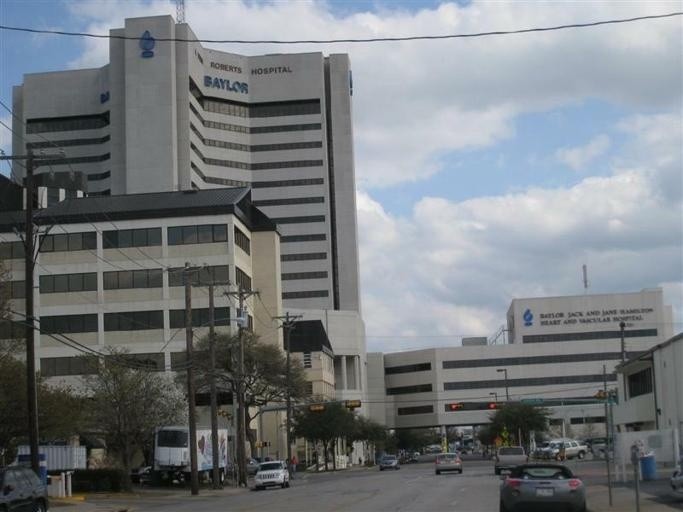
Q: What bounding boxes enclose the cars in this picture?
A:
[436,452,462,474]
[380,455,400,470]
[535,437,606,460]
[132,465,152,484]
[0,465,49,512]
[234,457,290,489]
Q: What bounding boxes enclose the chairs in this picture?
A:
[521,472,533,478]
[553,472,567,479]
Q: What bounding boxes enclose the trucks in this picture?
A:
[154,426,228,486]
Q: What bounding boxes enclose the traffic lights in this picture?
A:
[490,403,505,409]
[451,404,463,409]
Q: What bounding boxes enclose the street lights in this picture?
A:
[186,317,246,495]
[496,369,509,401]
[490,392,498,404]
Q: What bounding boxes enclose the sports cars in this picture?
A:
[500,463,586,511]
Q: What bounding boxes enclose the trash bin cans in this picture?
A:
[640,455,656,480]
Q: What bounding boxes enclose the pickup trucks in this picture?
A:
[495,445,527,475]
